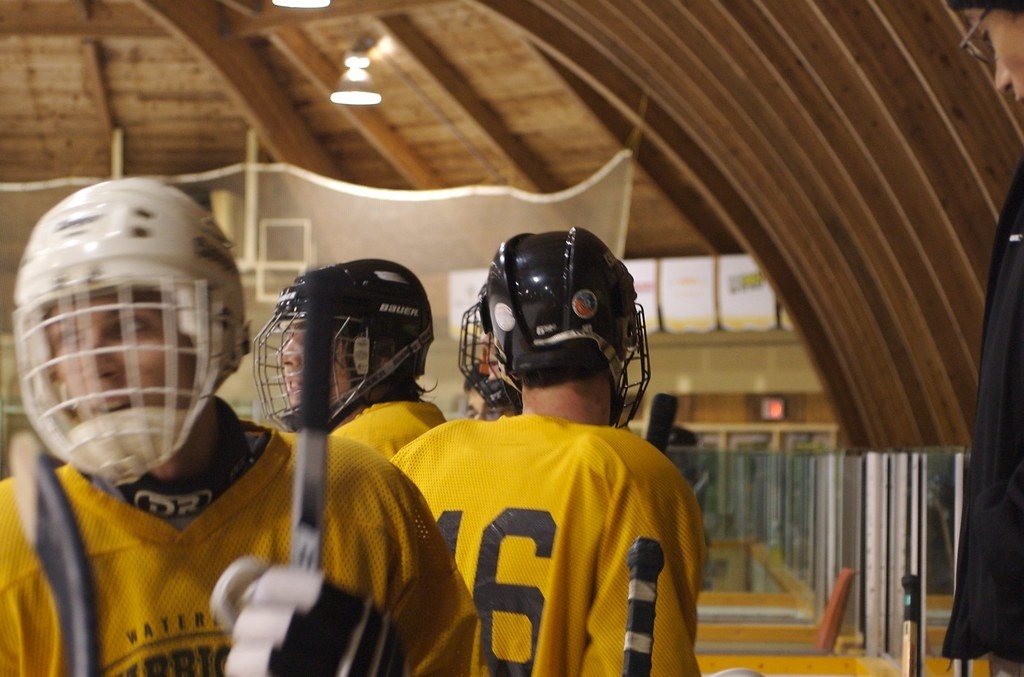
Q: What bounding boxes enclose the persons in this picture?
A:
[1,178,487,677]
[386,226,706,677]
[936,3,1024,677]
[465,361,523,422]
[255,258,447,460]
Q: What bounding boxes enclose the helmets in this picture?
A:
[275,258,436,429]
[480,225,652,428]
[13,176,250,483]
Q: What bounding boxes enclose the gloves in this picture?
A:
[207,556,405,677]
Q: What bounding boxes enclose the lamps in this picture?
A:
[330,48,382,105]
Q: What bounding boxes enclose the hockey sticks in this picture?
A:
[9,429,99,677]
[291,296,331,569]
[647,393,679,454]
[623,536,665,677]
